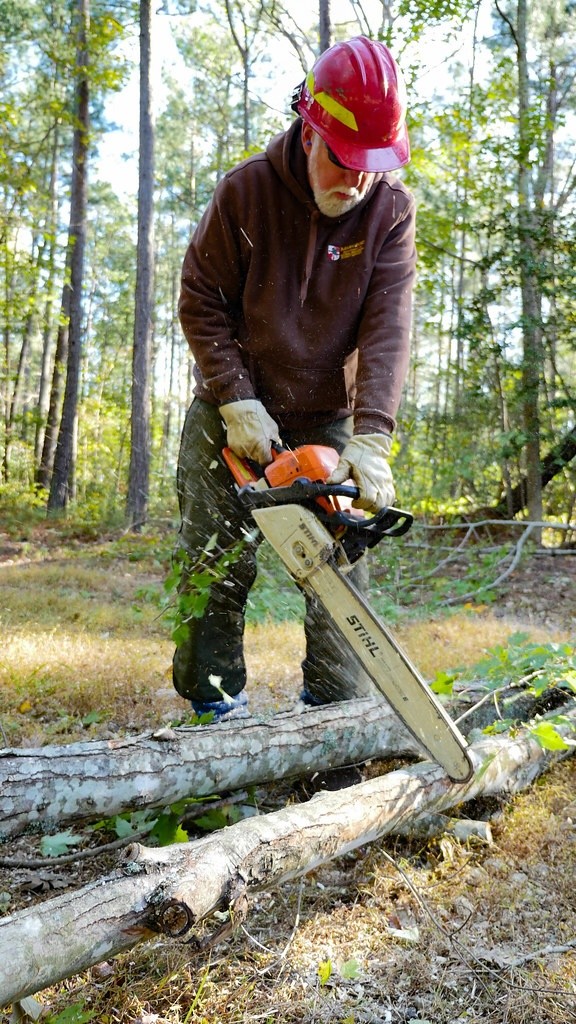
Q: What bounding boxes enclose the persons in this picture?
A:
[167,32,416,727]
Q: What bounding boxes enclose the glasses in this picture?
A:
[325,142,352,171]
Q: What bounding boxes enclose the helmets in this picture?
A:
[295,37,411,174]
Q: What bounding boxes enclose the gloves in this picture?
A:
[327,435,397,513]
[218,397,283,465]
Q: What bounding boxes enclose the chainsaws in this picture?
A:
[221,437,474,784]
